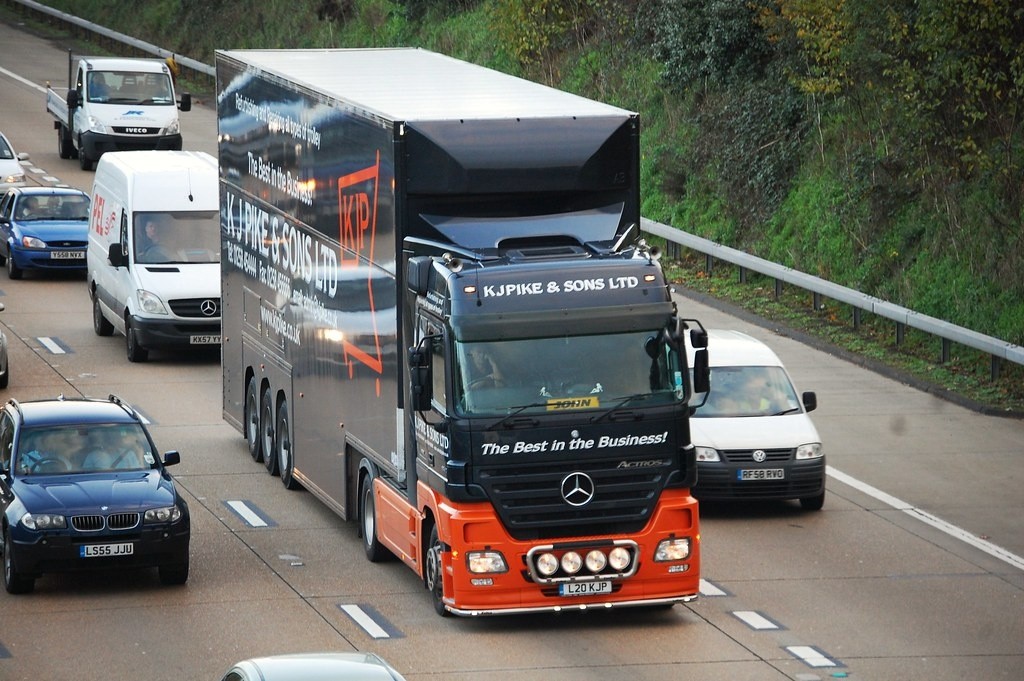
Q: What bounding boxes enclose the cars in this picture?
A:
[0,131,30,189]
[0,187,94,279]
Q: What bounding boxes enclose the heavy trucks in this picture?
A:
[214,46,712,618]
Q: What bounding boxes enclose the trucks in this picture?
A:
[46,48,192,169]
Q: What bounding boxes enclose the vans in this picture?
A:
[663,329,827,511]
[87,150,226,362]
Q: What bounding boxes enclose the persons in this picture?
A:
[460,344,506,388]
[137,216,181,261]
[90,72,118,98]
[536,336,618,396]
[22,196,53,219]
[3,431,72,474]
[82,427,145,471]
[740,375,775,412]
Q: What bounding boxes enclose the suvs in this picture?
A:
[0,392,190,593]
